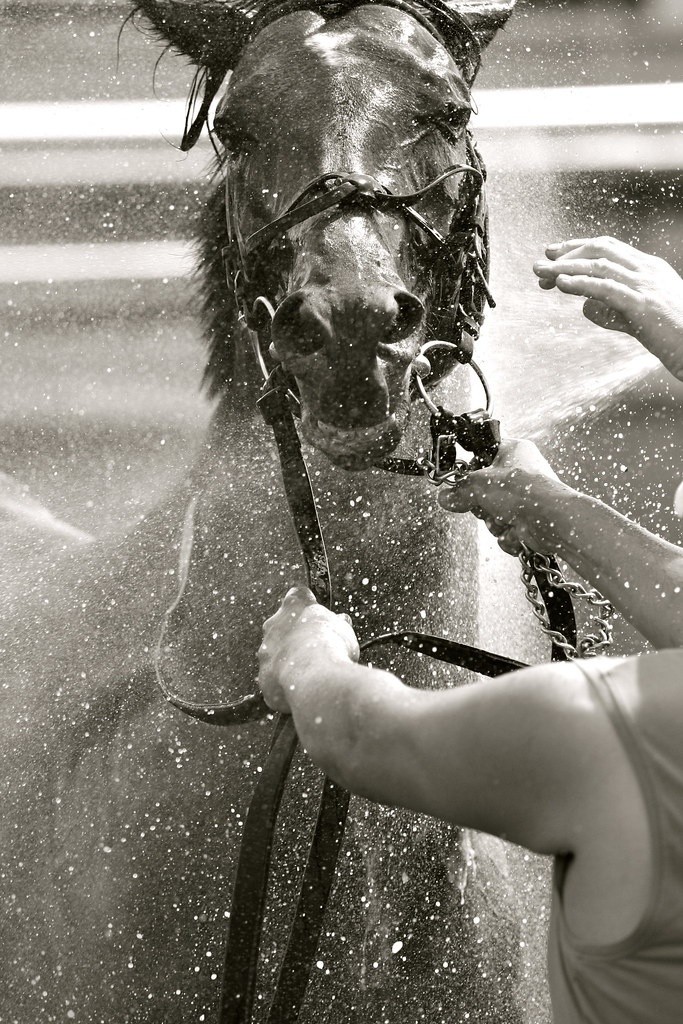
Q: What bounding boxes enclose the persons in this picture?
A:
[533,235,683,384]
[255,431,683,1017]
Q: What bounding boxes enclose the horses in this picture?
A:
[0,0,561,1023]
